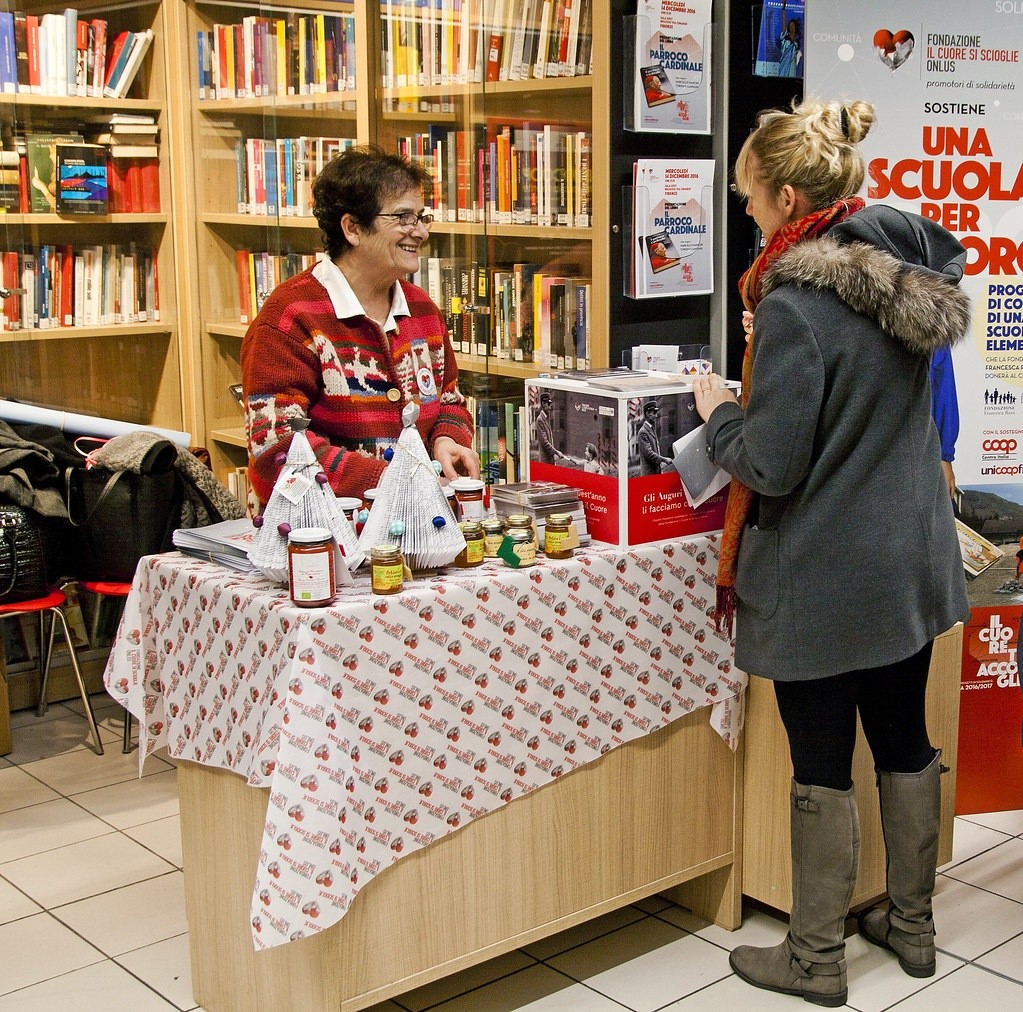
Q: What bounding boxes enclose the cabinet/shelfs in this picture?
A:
[0,1,612,514]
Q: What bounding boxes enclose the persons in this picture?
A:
[692,98,969,1007]
[638,400,673,477]
[239,145,482,528]
[536,393,605,475]
[929,344,959,499]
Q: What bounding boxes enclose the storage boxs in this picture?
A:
[525,375,742,549]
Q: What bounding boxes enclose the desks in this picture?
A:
[100,531,747,1012]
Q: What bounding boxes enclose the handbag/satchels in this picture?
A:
[0,505,50,601]
[60,466,175,585]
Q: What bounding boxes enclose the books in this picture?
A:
[238,136,358,217]
[490,480,592,548]
[407,250,591,370]
[229,384,244,408]
[0,8,155,99]
[227,465,249,509]
[199,11,355,99]
[455,373,527,489]
[0,111,160,214]
[558,369,687,392]
[0,237,159,331]
[381,0,711,134]
[235,248,327,326]
[396,118,714,298]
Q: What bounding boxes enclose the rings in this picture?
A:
[704,387,710,391]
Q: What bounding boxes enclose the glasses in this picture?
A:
[372,212,434,232]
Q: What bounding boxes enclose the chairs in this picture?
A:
[40,438,248,755]
[0,420,104,755]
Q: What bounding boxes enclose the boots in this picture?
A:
[857,747,949,978]
[729,777,860,1008]
